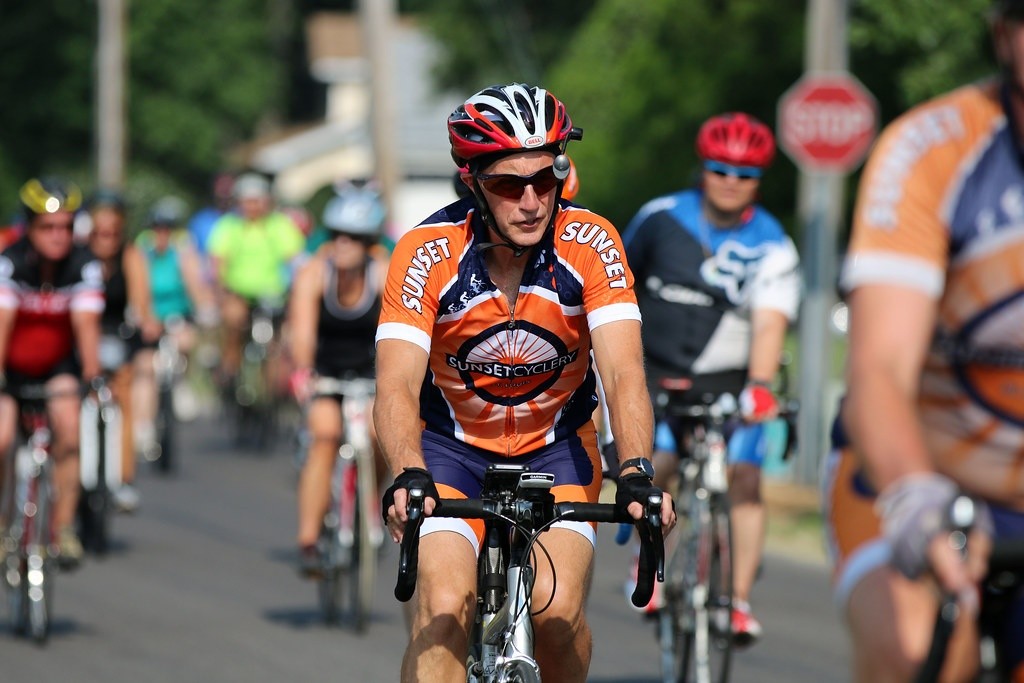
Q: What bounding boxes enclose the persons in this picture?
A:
[288,194,398,578]
[619,112,801,645]
[0,174,306,567]
[376,80,678,683]
[830,0,1024,683]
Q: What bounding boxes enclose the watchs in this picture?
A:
[620,457,655,481]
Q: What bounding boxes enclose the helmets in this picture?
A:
[697,110,775,166]
[321,186,390,236]
[19,174,88,218]
[447,83,574,170]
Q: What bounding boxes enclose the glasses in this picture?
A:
[476,163,563,199]
[709,165,761,181]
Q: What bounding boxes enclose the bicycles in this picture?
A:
[650,400,799,683]
[292,375,389,634]
[388,476,681,683]
[0,308,207,646]
[910,495,1024,683]
[213,286,299,454]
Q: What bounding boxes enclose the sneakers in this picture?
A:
[732,593,763,642]
[626,557,666,609]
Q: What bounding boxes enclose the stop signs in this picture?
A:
[775,71,876,185]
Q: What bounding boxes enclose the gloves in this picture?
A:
[735,385,780,427]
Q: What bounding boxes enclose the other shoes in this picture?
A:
[52,526,83,573]
[299,546,328,581]
